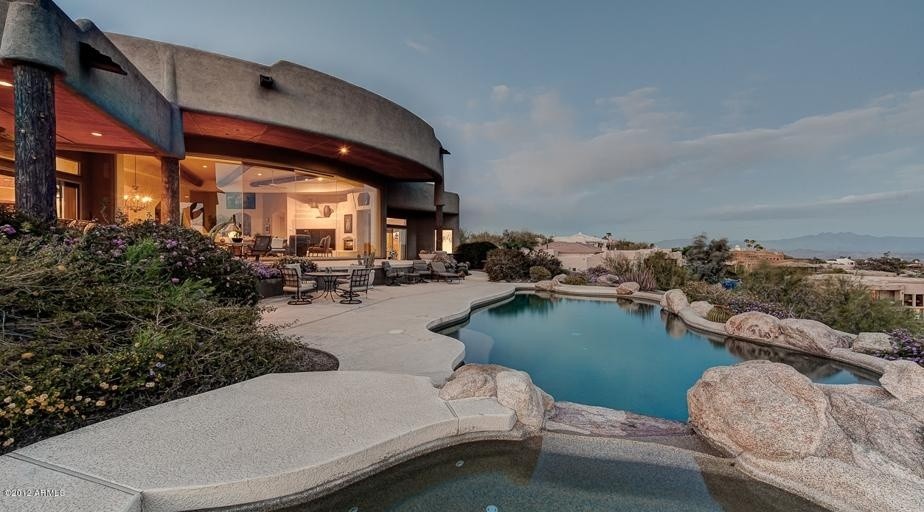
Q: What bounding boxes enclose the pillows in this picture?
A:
[320,238,326,248]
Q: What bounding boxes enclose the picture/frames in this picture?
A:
[344,214,352,233]
[180,201,204,226]
[226,192,256,209]
[344,239,353,250]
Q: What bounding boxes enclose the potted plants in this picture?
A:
[348,250,376,290]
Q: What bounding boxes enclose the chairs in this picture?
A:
[430,261,463,284]
[279,263,317,305]
[337,268,375,304]
[308,235,331,257]
[271,238,286,258]
[247,234,272,263]
[382,260,404,287]
[413,260,440,283]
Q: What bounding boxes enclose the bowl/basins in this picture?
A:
[232,238,243,242]
[419,254,436,260]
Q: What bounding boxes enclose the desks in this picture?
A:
[304,272,351,302]
[404,272,420,285]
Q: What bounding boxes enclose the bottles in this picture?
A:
[329,267,332,273]
[325,267,328,273]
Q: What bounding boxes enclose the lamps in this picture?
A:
[123,155,150,212]
